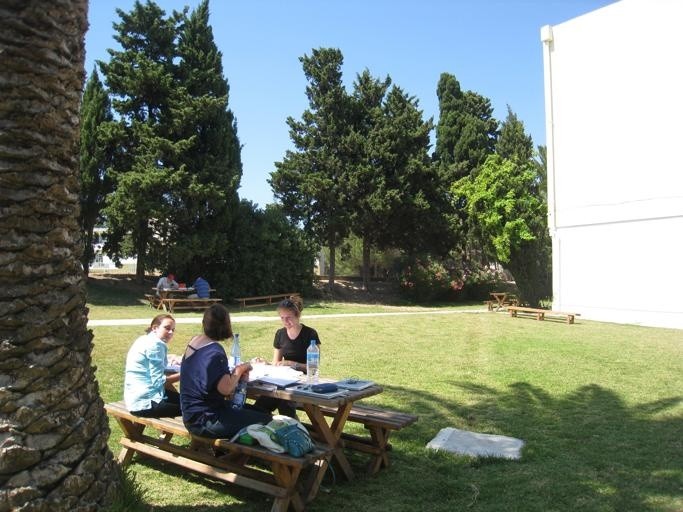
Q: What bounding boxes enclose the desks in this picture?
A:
[489,292,516,312]
[151,287,216,313]
[158,368,383,504]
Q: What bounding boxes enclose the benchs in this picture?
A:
[504,305,581,325]
[296,403,419,476]
[233,292,300,310]
[143,293,222,313]
[483,300,517,310]
[103,398,335,511]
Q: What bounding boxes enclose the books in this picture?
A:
[165,354,376,400]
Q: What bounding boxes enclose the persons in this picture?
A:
[180,303,272,440]
[253,295,321,423]
[124,314,181,417]
[156,272,211,304]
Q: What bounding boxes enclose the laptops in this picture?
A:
[285,384,349,399]
[335,380,375,391]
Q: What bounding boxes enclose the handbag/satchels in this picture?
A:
[270,424,316,458]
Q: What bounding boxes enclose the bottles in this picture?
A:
[230,333,241,368]
[307,339,320,384]
[229,374,249,412]
